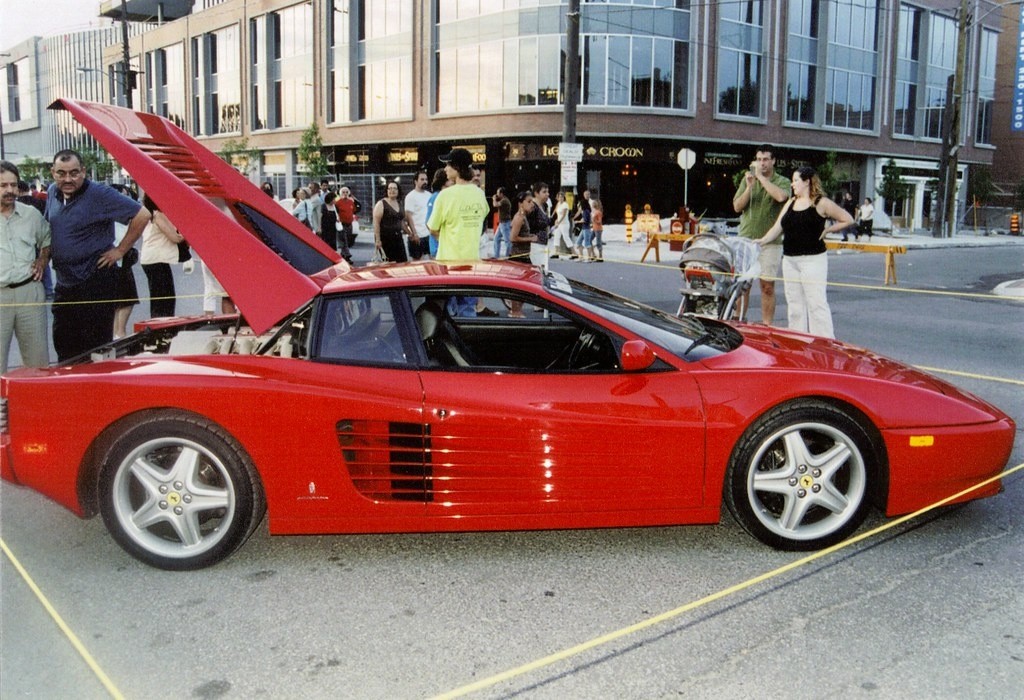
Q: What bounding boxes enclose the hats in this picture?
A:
[438,148,473,168]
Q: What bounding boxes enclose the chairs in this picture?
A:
[415,297,487,367]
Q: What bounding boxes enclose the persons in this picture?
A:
[262,146,874,343]
[0,150,239,376]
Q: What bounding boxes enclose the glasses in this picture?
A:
[388,187,398,191]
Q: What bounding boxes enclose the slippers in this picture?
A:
[476,306,499,317]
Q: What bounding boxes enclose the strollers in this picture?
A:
[677,231,760,322]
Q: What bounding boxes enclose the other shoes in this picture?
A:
[346,254,352,258]
[568,256,579,260]
[582,259,592,263]
[507,312,526,319]
[346,258,353,265]
[550,255,559,258]
[574,258,585,262]
[592,258,603,262]
[182,259,194,275]
[841,239,848,241]
[534,306,552,313]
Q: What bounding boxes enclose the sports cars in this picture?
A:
[0,98,1018,572]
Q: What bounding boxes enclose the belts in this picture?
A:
[7,275,36,289]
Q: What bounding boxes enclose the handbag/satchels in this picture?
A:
[300,201,313,230]
[335,221,343,232]
[367,247,395,267]
